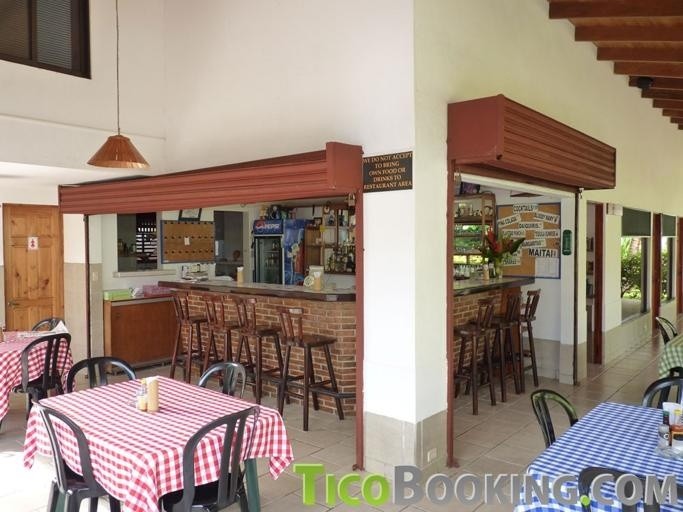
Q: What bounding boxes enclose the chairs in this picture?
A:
[168,289,343,431]
[32,318,65,332]
[452,288,540,415]
[656,316,677,345]
[531,389,578,449]
[30,398,120,512]
[158,405,260,512]
[67,356,136,393]
[11,333,71,422]
[578,466,683,511]
[642,366,683,410]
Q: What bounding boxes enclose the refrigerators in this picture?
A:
[253,217,315,286]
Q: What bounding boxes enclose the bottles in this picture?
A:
[325,245,355,274]
[658,409,683,453]
[453,199,499,281]
[133,376,162,411]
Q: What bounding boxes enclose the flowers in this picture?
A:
[478,228,526,264]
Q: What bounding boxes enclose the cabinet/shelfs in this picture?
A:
[304,202,356,275]
[454,190,497,280]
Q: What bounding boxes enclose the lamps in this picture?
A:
[87,2,151,170]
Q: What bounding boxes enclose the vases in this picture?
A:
[493,256,504,279]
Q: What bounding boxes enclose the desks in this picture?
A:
[0,332,78,428]
[103,294,180,376]
[658,333,683,380]
[23,375,295,512]
[511,401,683,512]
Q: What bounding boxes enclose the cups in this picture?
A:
[313,278,323,290]
[237,270,244,283]
